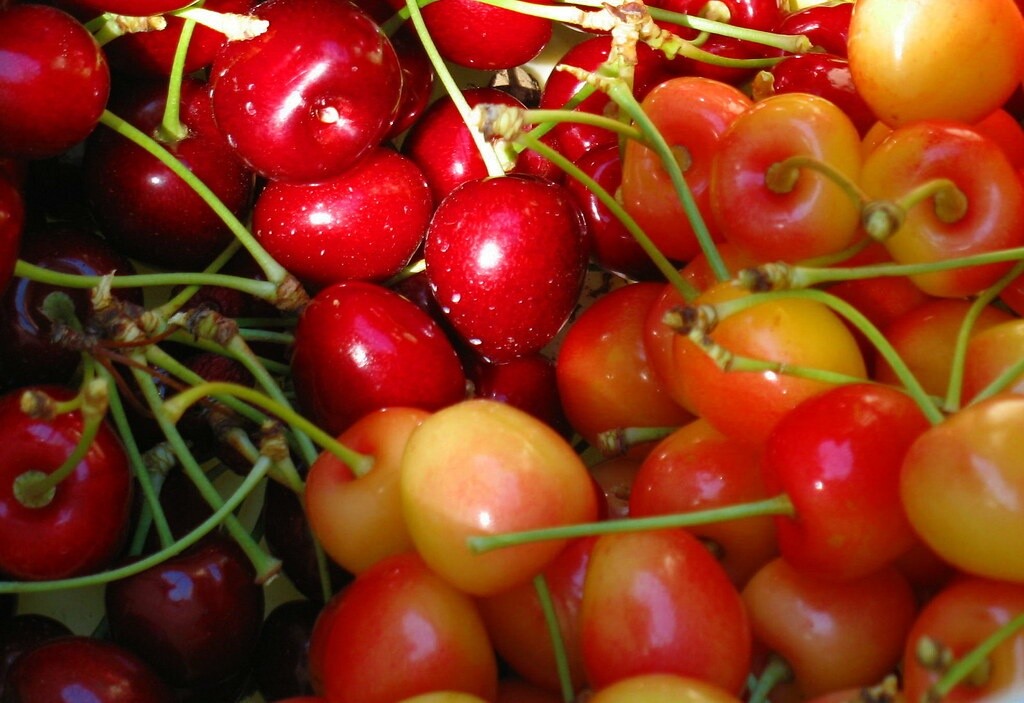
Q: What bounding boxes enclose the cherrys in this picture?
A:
[1,0,1023,703]
